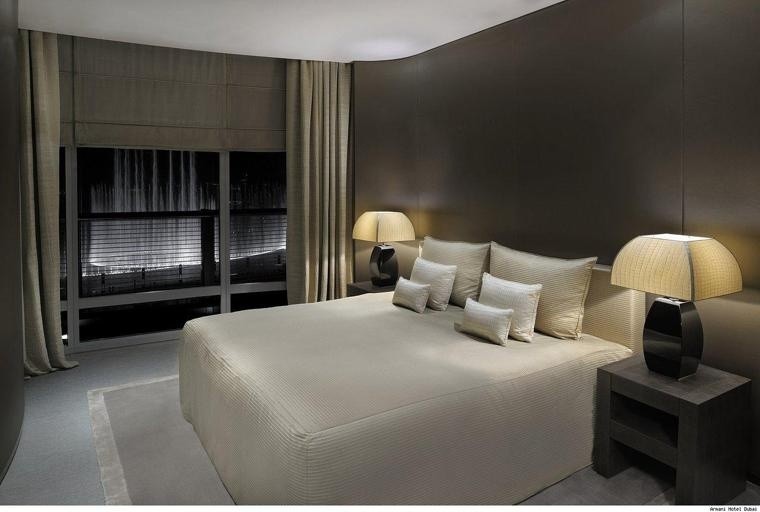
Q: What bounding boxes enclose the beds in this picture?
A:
[180,236,646,504]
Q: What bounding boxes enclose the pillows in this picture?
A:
[477,272,543,343]
[392,276,431,313]
[409,256,457,311]
[489,241,598,340]
[420,235,490,308]
[458,298,514,345]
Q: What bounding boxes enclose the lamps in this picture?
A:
[610,233,743,379]
[352,211,416,287]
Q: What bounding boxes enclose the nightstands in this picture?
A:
[347,280,397,300]
[594,352,751,505]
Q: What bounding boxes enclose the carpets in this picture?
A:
[85,374,760,505]
